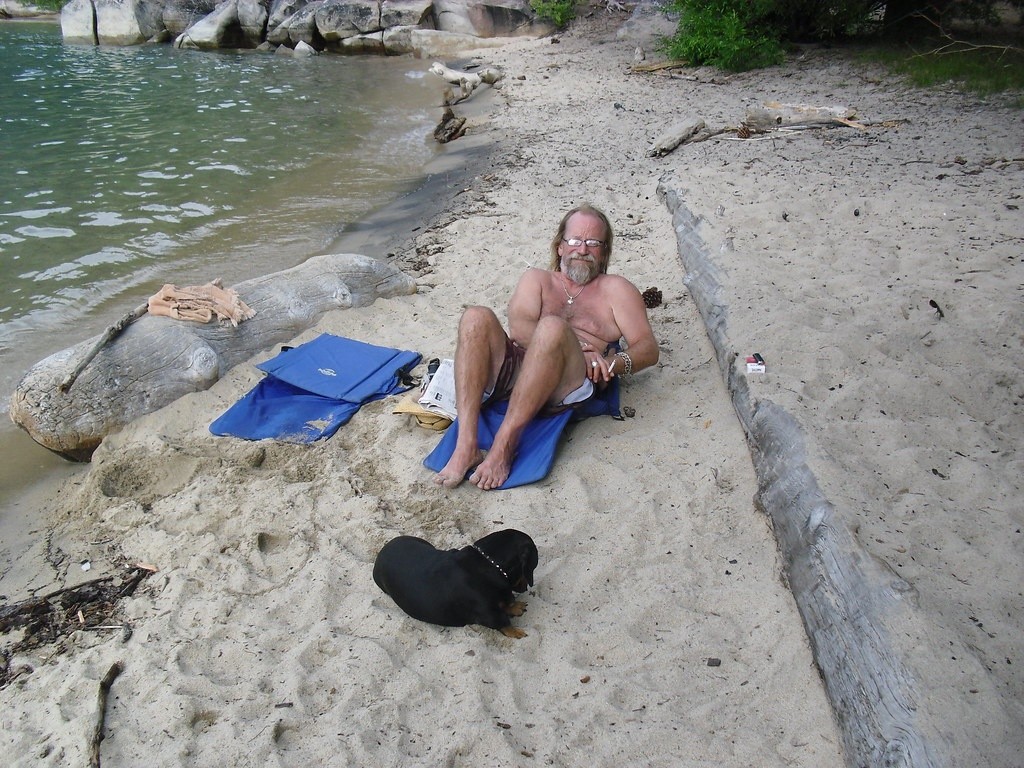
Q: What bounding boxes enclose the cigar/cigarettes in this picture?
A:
[607,358,617,376]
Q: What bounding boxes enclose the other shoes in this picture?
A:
[418,357,441,399]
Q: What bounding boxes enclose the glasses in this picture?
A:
[561,237,608,248]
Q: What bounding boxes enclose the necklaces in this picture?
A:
[560,278,585,304]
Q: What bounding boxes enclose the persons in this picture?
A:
[432,203,661,491]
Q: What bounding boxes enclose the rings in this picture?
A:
[592,361,599,367]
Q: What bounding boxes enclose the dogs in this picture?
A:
[372,528,539,640]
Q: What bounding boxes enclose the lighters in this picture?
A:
[753,352,765,366]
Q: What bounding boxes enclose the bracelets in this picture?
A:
[616,352,632,379]
